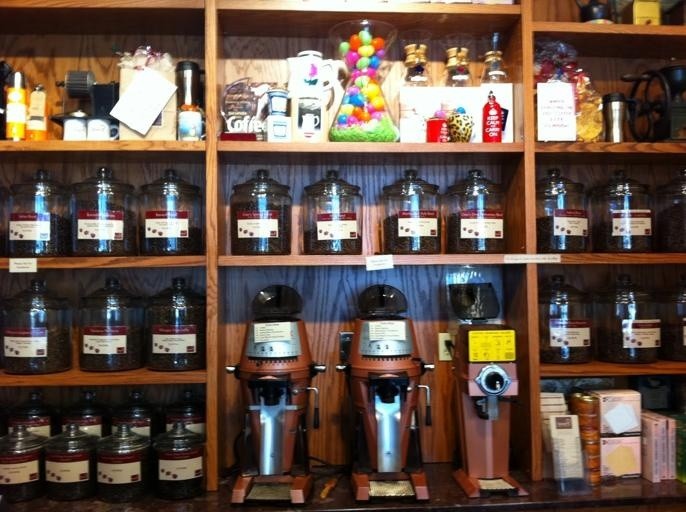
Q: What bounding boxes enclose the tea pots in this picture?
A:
[287,49,339,94]
[301,113,320,131]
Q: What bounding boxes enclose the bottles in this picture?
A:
[401,29,510,86]
[229,167,504,256]
[144,273,206,372]
[1,276,73,375]
[6,70,48,142]
[481,91,503,142]
[534,165,686,363]
[137,165,206,257]
[70,162,138,256]
[327,18,400,142]
[2,390,204,504]
[2,164,70,256]
[78,274,144,373]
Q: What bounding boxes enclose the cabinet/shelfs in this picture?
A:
[212,3,527,512]
[0,2,211,512]
[532,1,686,508]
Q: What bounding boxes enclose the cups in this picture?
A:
[425,114,474,142]
[602,92,626,142]
[62,118,119,141]
[177,110,207,141]
[267,89,288,116]
[273,121,287,138]
[175,61,200,106]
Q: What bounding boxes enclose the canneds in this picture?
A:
[571,393,603,488]
